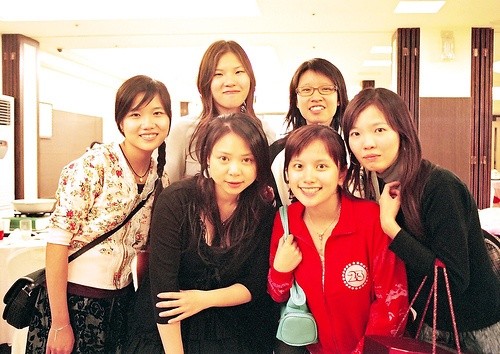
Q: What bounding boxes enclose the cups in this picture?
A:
[0,222,5,240]
[20,219,32,239]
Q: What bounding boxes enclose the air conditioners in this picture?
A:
[0,94,15,220]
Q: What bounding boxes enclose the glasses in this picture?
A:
[295,85,338,96]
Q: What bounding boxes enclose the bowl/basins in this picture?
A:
[12,199,56,213]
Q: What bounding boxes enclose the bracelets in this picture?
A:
[48,321,71,343]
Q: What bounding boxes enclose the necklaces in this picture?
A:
[208,200,239,225]
[117,143,152,184]
[306,207,341,251]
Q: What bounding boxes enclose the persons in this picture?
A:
[25,75,171,354]
[164,38,278,193]
[343,86,500,354]
[124,113,273,354]
[267,58,371,207]
[266,124,409,354]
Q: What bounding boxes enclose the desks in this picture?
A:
[0,233,47,354]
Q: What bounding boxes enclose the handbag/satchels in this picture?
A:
[275,204,319,348]
[360,266,462,353]
[2,268,45,330]
